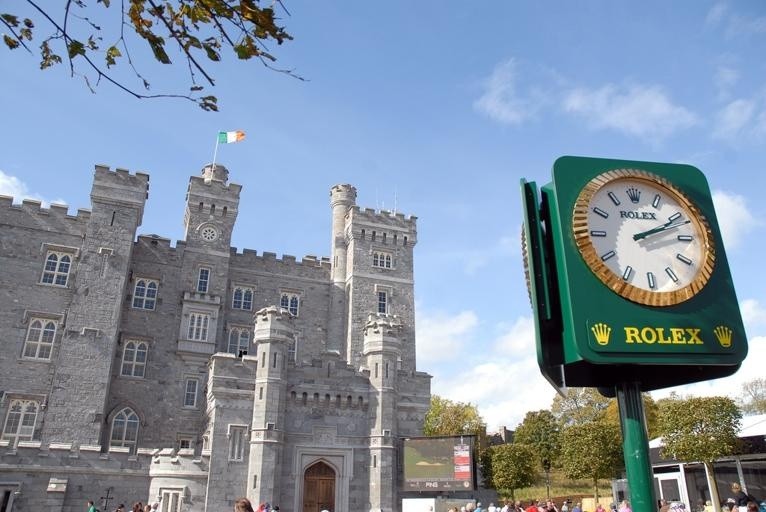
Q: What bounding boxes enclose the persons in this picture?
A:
[448,497,766,511]
[115,502,160,512]
[255,502,281,512]
[87,499,96,512]
[233,497,254,511]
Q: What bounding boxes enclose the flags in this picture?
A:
[218,129,245,145]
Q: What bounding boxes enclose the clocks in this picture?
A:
[573,169,717,307]
